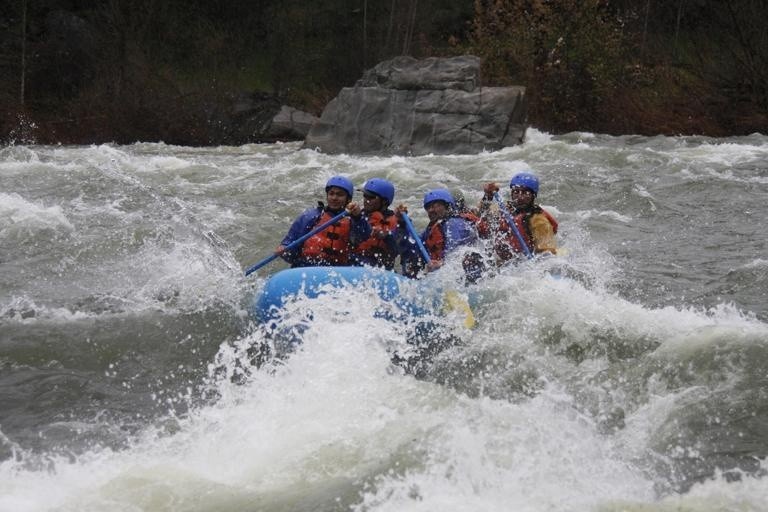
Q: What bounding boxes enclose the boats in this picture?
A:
[254,258,594,334]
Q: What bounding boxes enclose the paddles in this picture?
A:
[402,211,475,328]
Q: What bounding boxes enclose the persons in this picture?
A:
[347,177,412,271]
[447,188,489,240]
[274,174,372,268]
[478,172,559,256]
[393,188,486,287]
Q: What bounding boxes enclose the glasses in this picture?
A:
[511,189,532,195]
[363,193,376,200]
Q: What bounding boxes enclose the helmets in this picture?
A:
[448,188,465,208]
[364,178,394,205]
[510,172,538,192]
[325,175,353,196]
[424,189,455,208]
